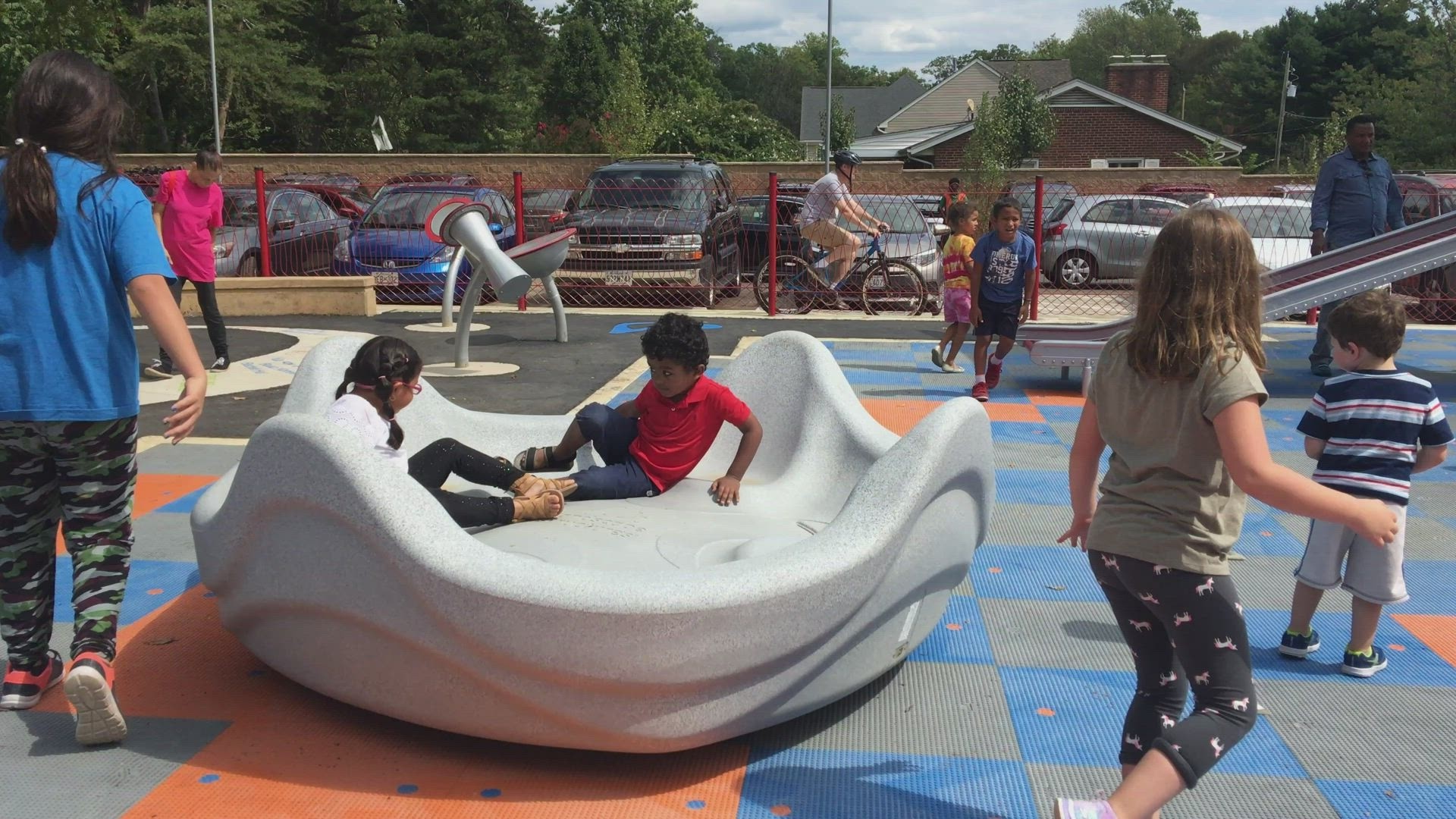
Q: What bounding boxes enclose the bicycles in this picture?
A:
[752,225,929,317]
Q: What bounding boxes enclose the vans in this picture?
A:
[988,179,1079,250]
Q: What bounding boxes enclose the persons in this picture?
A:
[1309,114,1406,376]
[517,313,764,505]
[796,149,890,292]
[932,177,1037,401]
[1049,207,1401,817]
[1278,288,1456,679]
[0,49,210,745]
[144,137,230,379]
[325,334,579,528]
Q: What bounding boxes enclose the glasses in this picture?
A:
[392,381,422,394]
[1358,159,1373,179]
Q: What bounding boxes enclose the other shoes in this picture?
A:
[931,345,944,367]
[1054,797,1118,819]
[1310,363,1332,377]
[942,364,965,373]
[836,300,850,309]
[810,262,825,288]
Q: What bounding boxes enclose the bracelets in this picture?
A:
[1023,298,1032,301]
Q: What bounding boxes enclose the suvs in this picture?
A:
[534,153,743,307]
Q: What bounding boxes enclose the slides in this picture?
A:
[1015,209,1456,367]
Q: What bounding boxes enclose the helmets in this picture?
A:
[833,150,862,165]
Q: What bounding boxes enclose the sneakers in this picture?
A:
[985,352,1002,389]
[144,359,173,378]
[972,382,989,400]
[0,649,66,710]
[1279,624,1321,657]
[210,356,231,372]
[1341,644,1388,677]
[62,650,128,745]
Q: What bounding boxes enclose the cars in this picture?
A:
[1190,195,1329,274]
[266,167,945,307]
[1125,180,1217,213]
[816,191,944,317]
[1041,192,1190,290]
[1264,167,1456,324]
[329,184,516,307]
[208,183,361,278]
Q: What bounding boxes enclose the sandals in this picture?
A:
[513,445,577,472]
[504,473,578,498]
[510,489,564,523]
